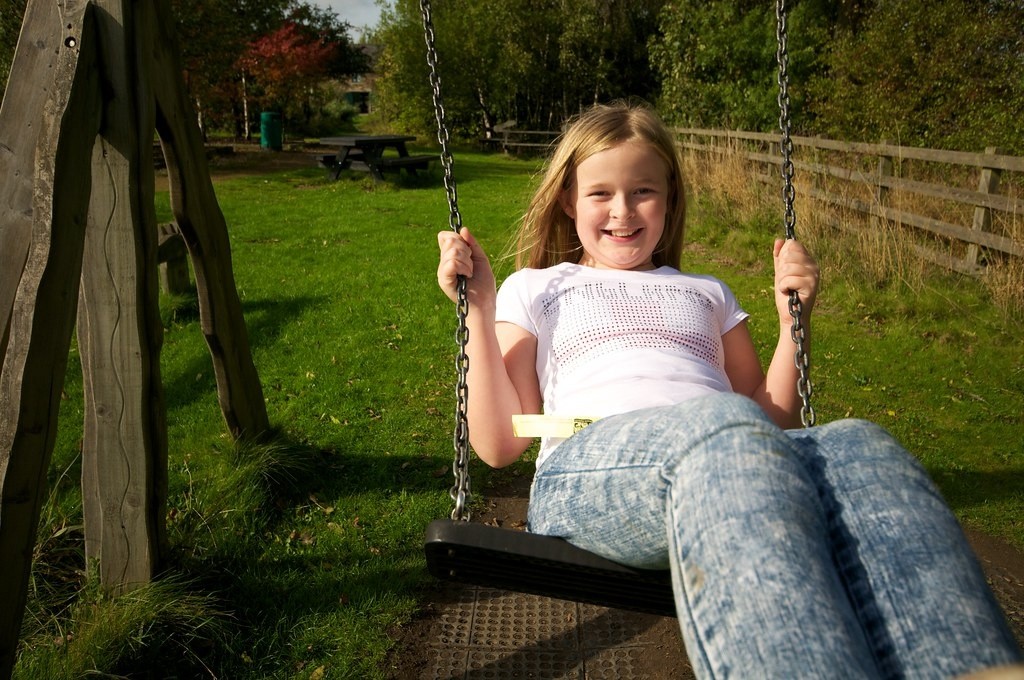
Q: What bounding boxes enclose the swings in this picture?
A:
[411,0,822,620]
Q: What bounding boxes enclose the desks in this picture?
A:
[317,136,420,181]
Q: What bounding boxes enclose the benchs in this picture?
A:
[316,152,365,163]
[366,154,440,165]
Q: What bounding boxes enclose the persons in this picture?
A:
[435,102,1024,679]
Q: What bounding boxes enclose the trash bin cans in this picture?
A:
[260,111,283,153]
